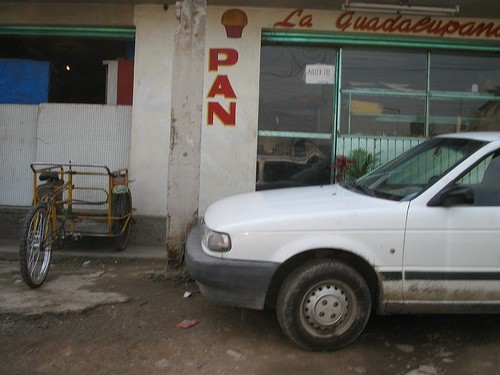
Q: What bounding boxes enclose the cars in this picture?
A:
[185,132,500,351]
[257,151,331,190]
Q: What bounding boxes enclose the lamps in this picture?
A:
[341,0,460,18]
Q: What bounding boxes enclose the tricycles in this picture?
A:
[19,160,137,289]
